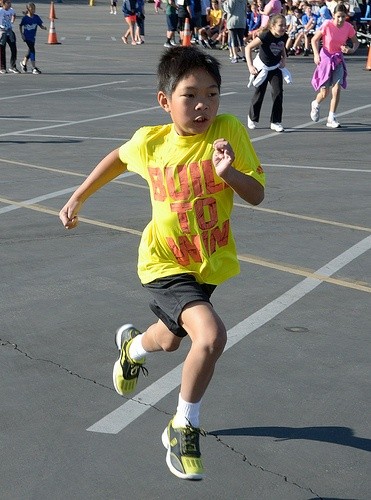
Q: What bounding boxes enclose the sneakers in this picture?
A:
[161,415,207,482]
[112,324,148,397]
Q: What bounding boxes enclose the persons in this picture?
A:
[310,4,360,128]
[282,0,361,56]
[58,47,265,481]
[362,0,371,18]
[19,2,47,74]
[109,0,118,15]
[0,0,21,74]
[121,0,146,45]
[223,0,287,63]
[245,14,286,131]
[153,0,166,15]
[165,0,229,50]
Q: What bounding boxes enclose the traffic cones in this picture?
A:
[44,18,62,45]
[362,43,371,71]
[88,0,96,6]
[47,1,58,19]
[182,18,191,47]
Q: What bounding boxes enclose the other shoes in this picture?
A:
[0,69,7,74]
[193,37,230,51]
[270,122,284,133]
[31,67,41,75]
[311,101,320,122]
[326,117,341,128]
[170,40,177,46]
[231,58,238,64]
[9,68,20,74]
[121,36,145,46]
[284,46,313,57]
[164,43,173,48]
[242,56,246,61]
[20,60,27,72]
[247,115,256,130]
